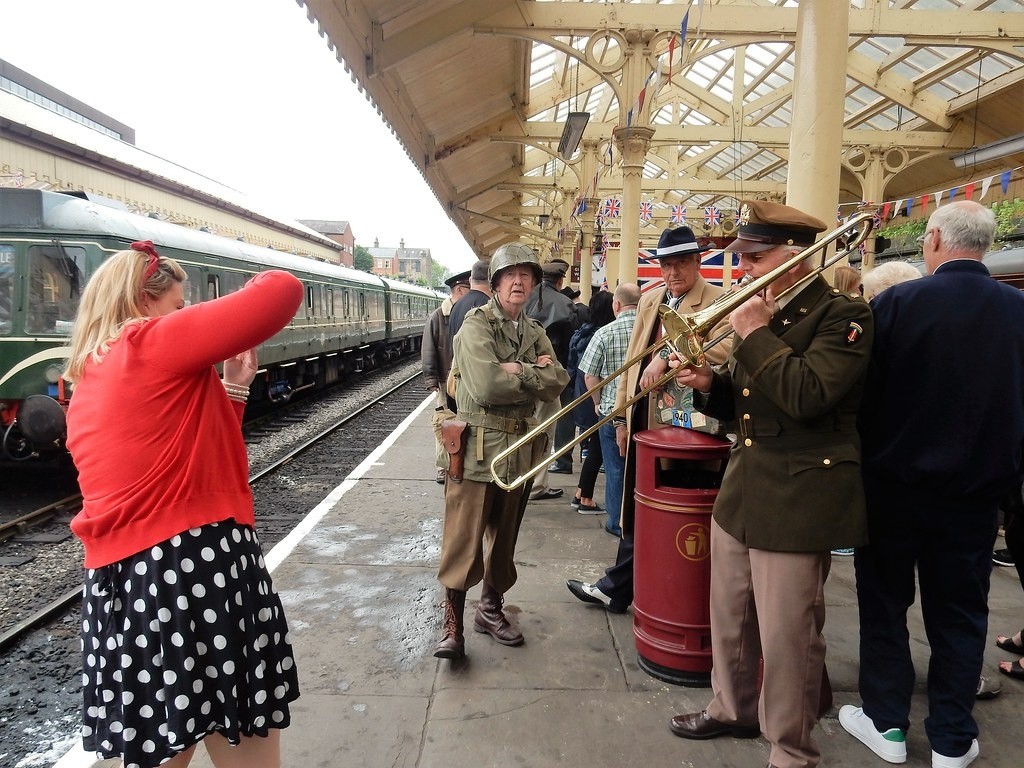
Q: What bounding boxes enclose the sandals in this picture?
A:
[996,628,1024,656]
[999,660,1024,681]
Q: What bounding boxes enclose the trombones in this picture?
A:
[490,212,874,493]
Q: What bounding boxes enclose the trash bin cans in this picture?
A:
[631,426,734,687]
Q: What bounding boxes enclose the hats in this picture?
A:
[543,263,569,278]
[725,199,827,253]
[560,286,581,299]
[643,225,711,260]
[471,259,490,280]
[444,270,472,289]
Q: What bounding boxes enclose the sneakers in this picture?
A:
[976,674,1001,700]
[992,549,1015,566]
[571,497,581,508]
[577,502,608,514]
[838,705,907,763]
[932,738,979,768]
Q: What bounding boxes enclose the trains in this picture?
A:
[0,189,448,508]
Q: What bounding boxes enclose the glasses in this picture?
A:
[916,227,940,247]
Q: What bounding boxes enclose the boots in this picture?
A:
[474,582,524,645]
[433,588,466,660]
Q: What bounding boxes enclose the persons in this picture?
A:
[423,226,1023,720]
[838,197,1024,767]
[671,197,871,768]
[61,241,304,767]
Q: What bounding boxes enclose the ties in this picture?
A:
[662,297,679,337]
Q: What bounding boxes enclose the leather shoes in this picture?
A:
[436,469,445,483]
[669,709,762,740]
[528,488,563,501]
[566,579,629,614]
[548,464,573,474]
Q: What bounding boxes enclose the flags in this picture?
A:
[593,197,739,292]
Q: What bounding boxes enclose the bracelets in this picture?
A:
[613,420,625,428]
[220,379,249,404]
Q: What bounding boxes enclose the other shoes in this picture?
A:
[831,548,854,555]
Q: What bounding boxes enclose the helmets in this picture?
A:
[488,241,543,293]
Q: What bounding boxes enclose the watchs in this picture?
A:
[659,348,672,362]
